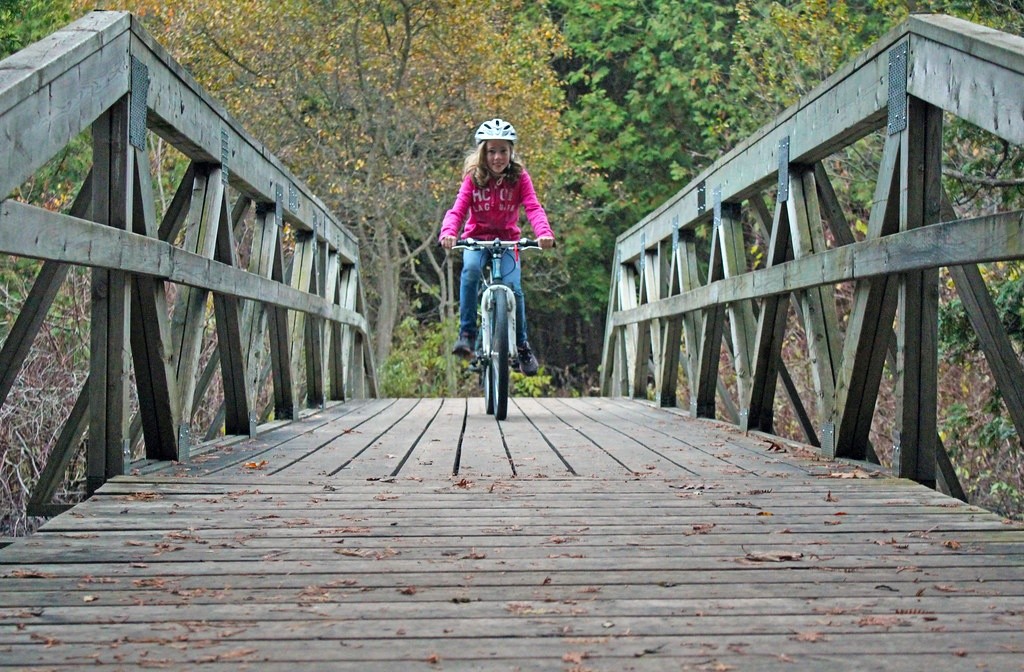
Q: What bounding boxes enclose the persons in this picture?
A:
[437,119,555,376]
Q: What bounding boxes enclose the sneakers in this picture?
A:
[517,341,538,376]
[451,333,475,359]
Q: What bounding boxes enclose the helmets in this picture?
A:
[474,119,517,144]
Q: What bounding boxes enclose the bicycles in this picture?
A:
[437,237,558,420]
